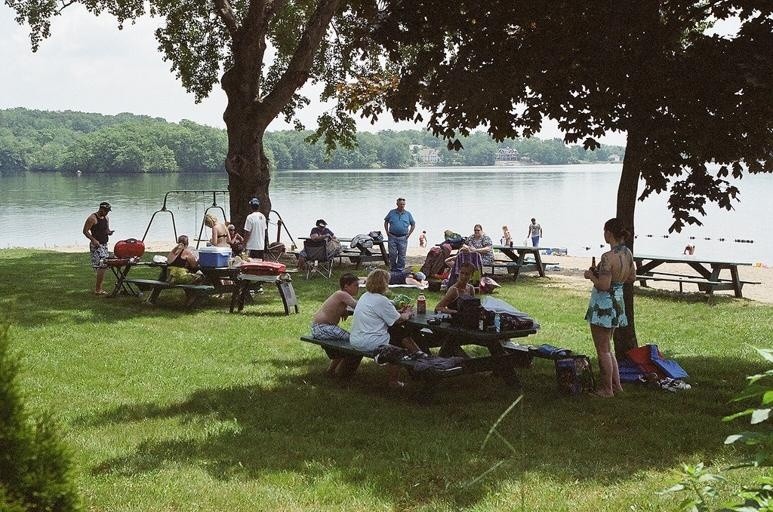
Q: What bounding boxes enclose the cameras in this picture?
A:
[427,317,441,325]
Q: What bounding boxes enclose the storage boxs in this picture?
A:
[199,245,232,268]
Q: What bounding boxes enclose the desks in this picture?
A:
[227,273,298,316]
[632,255,753,298]
[104,257,141,299]
[134,258,255,314]
[351,305,536,388]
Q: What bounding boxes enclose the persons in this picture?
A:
[349,269,429,392]
[527,218,542,247]
[584,217,637,398]
[435,261,476,314]
[683,242,695,255]
[433,224,494,279]
[384,198,416,271]
[83,202,115,295]
[501,225,512,246]
[311,272,359,373]
[419,230,427,246]
[298,218,335,270]
[167,197,268,296]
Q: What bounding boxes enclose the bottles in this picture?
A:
[477,309,502,335]
[508,237,514,247]
[414,293,426,314]
[500,236,505,247]
[227,252,232,268]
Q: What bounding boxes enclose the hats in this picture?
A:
[167,243,185,264]
[249,197,260,206]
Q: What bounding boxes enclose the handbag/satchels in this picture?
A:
[369,231,383,241]
[115,238,145,259]
[554,355,595,393]
[420,243,452,277]
[350,234,375,248]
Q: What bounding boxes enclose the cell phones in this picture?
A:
[110,229,115,233]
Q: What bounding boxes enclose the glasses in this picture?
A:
[101,205,111,208]
[398,199,405,201]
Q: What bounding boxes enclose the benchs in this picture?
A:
[266,235,561,282]
[471,337,540,367]
[299,335,463,377]
[648,270,762,291]
[634,274,722,304]
[121,278,215,308]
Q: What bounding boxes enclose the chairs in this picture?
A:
[440,251,484,294]
